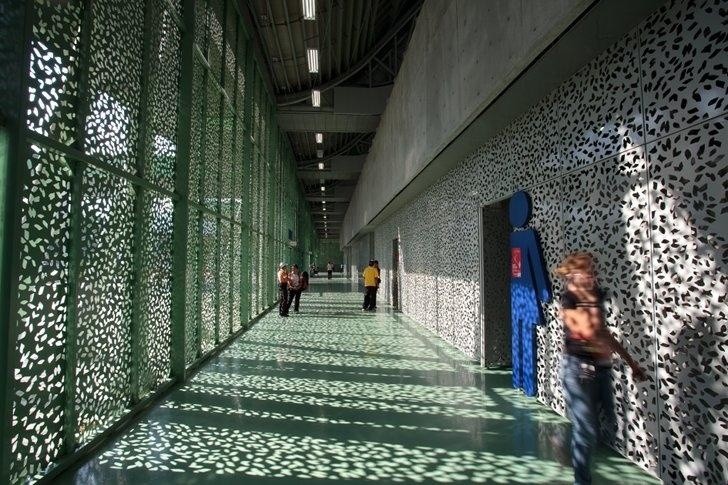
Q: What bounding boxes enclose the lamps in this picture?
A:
[306,45,318,74]
[310,86,321,109]
[315,131,324,145]
[300,0,317,22]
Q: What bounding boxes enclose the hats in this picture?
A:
[280,263,288,268]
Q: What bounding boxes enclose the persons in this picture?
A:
[553,254,641,485]
[277,260,291,317]
[361,258,381,312]
[370,261,382,308]
[286,264,304,316]
[309,263,344,276]
[326,261,333,280]
